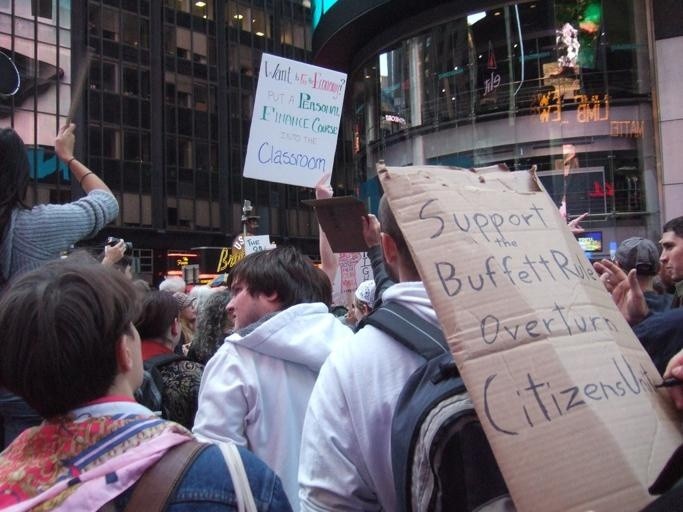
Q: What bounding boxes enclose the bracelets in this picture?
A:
[78,170,94,189]
[68,157,75,168]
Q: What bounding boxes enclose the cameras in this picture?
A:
[107,236,132,256]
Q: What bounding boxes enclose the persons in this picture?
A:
[566,209,683,420]
[0,193,517,511]
[314,172,340,287]
[0,123,119,275]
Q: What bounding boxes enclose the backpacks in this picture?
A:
[133,354,185,420]
[369,303,517,512]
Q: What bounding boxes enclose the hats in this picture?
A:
[612,237,660,274]
[356,279,376,309]
[173,292,196,310]
